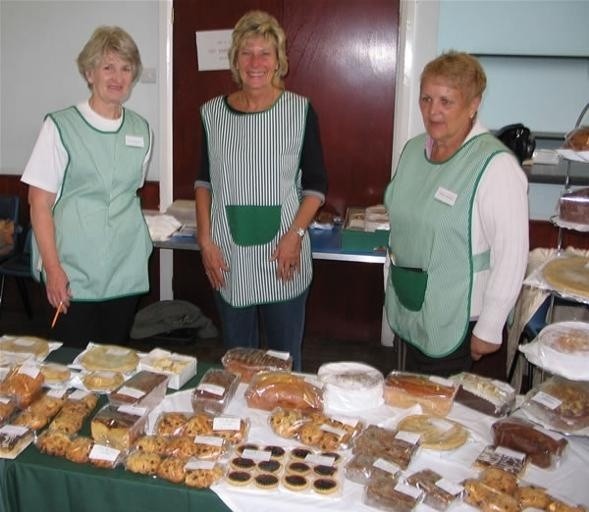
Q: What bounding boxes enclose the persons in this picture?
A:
[20,24,154,347]
[195,9,327,374]
[380,48,530,383]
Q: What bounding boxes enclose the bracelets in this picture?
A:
[291,224,306,239]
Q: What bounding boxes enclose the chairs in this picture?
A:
[0,227,31,320]
[0,196,22,263]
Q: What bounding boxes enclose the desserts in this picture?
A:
[0,321,589,512]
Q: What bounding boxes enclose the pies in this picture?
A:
[542,257,589,298]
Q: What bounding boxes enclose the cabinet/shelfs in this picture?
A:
[539,101,588,382]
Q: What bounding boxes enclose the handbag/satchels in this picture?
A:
[494,124,536,163]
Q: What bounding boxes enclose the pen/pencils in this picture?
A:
[51,300,63,329]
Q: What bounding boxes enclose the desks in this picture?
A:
[0,334,589,511]
[149,199,408,373]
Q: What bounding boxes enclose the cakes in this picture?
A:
[314,202,341,223]
[560,187,589,221]
[562,124,588,151]
